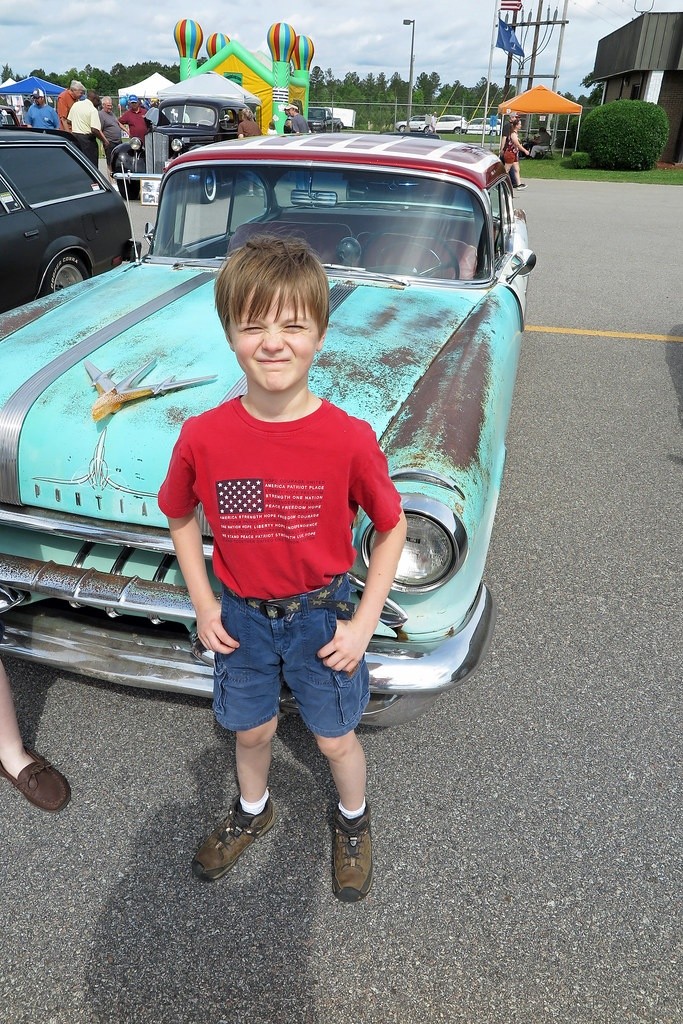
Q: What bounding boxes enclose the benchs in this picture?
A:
[279,213,474,244]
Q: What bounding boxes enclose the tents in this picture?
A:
[498,85,582,158]
[118,72,176,117]
[0,76,66,105]
[157,71,261,129]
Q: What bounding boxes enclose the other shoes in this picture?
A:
[245,193,253,197]
[516,183,528,190]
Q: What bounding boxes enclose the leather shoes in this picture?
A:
[0,746,70,814]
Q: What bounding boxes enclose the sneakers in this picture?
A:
[191,788,276,882]
[332,801,373,902]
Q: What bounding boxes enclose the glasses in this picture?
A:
[33,97,39,99]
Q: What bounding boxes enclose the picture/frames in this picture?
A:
[140,177,162,206]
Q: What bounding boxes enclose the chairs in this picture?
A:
[227,223,355,264]
[541,137,558,160]
[361,234,477,280]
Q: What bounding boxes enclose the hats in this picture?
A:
[129,95,138,103]
[30,88,44,97]
[509,111,521,118]
[285,104,298,109]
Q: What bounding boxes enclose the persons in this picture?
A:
[237,108,264,197]
[117,94,152,145]
[268,122,277,135]
[149,99,156,108]
[283,104,310,134]
[500,112,529,190]
[58,80,87,133]
[429,112,437,135]
[98,96,133,182]
[25,89,59,130]
[526,127,552,160]
[157,233,408,901]
[0,658,71,812]
[67,89,109,169]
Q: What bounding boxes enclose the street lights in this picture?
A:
[403,19,416,131]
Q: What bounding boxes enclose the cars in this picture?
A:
[0,129,537,728]
[109,94,259,204]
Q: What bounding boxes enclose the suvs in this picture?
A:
[0,130,142,314]
[396,115,425,134]
[465,118,501,136]
[418,115,468,134]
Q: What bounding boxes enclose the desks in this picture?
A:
[521,140,532,157]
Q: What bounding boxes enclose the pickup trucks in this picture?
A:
[308,106,342,132]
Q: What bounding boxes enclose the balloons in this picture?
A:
[120,98,126,107]
